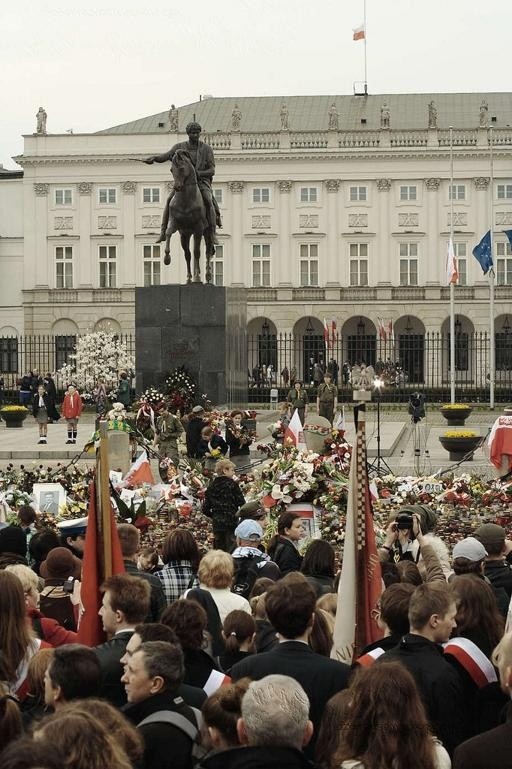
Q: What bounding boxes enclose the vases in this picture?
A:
[0,411,27,427]
[439,437,483,460]
[439,409,472,425]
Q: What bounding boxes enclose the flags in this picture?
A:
[353,27,366,41]
[446,230,460,288]
[472,229,492,277]
[376,317,395,348]
[322,317,337,348]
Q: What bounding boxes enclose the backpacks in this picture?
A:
[229,556,263,600]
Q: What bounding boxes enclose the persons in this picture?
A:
[286,373,339,429]
[427,100,439,127]
[328,102,338,128]
[16,367,134,445]
[168,104,179,130]
[139,123,223,246]
[247,356,409,388]
[36,106,47,133]
[232,104,242,130]
[151,402,253,485]
[2,458,512,769]
[279,104,289,128]
[381,102,390,126]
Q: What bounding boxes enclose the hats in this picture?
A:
[192,405,204,413]
[39,546,82,580]
[233,518,264,542]
[452,537,489,562]
[54,516,87,539]
[233,499,267,520]
[323,372,333,378]
[294,379,303,385]
[472,522,507,545]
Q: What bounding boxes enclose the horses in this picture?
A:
[164,149,212,285]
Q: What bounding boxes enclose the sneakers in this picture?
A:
[66,440,76,444]
[38,439,47,444]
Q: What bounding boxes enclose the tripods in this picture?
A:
[396,422,433,477]
[367,393,394,478]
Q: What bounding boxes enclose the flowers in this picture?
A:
[1,405,28,411]
[444,430,477,439]
[442,403,470,410]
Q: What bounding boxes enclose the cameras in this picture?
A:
[63,576,74,594]
[395,513,414,530]
